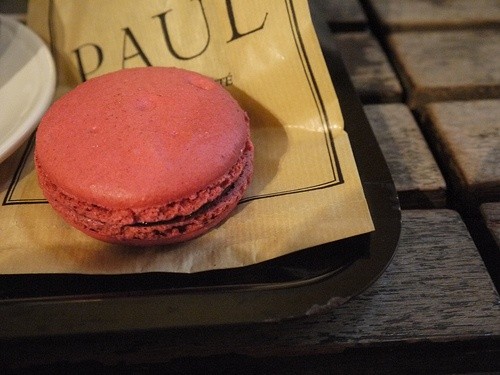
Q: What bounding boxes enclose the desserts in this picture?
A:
[35,66,256,248]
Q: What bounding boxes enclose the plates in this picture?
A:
[1,15,57,162]
